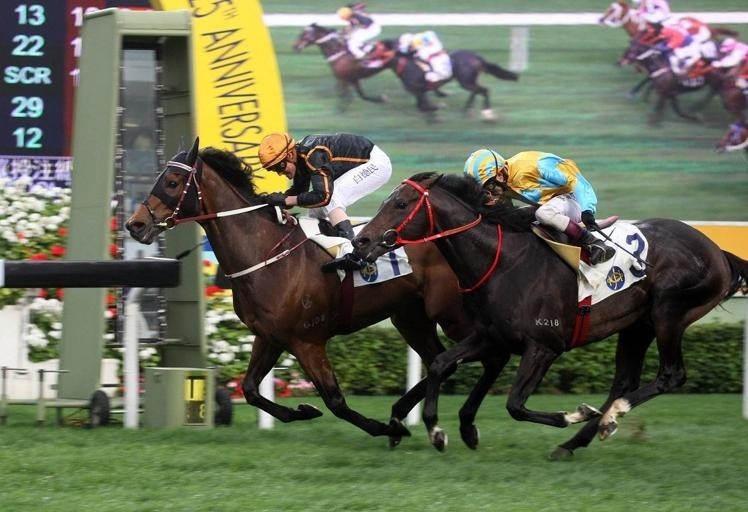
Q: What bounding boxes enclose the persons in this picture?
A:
[462,147,617,267]
[645,15,704,82]
[662,11,715,66]
[397,27,454,85]
[632,0,673,39]
[333,4,382,68]
[709,37,748,112]
[254,130,393,273]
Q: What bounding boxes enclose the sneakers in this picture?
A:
[589,239,616,266]
[322,254,366,272]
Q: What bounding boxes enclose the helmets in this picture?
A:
[258,129,297,173]
[336,6,352,20]
[462,146,509,189]
[397,30,416,55]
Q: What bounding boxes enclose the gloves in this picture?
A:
[263,191,291,210]
[581,210,598,233]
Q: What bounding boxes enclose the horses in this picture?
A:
[361,38,521,119]
[124,134,512,453]
[350,170,747,462]
[290,22,450,104]
[598,0,748,153]
